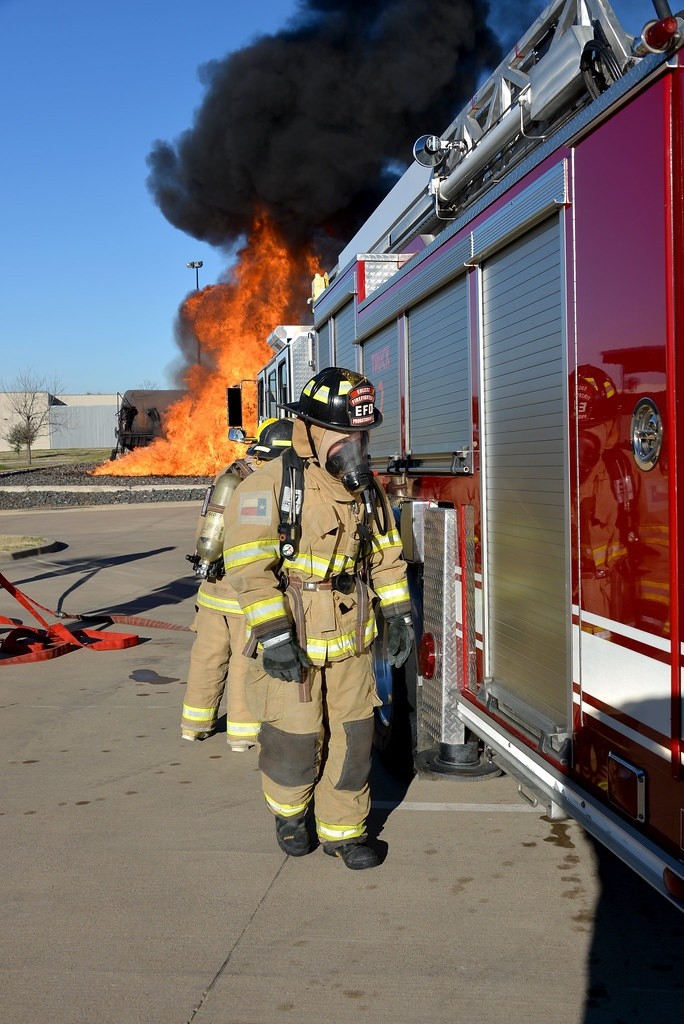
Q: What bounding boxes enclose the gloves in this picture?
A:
[262,641,310,683]
[386,613,414,667]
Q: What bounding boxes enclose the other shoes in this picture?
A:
[230,743,249,752]
[181,727,200,741]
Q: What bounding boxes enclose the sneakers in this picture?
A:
[274,814,311,856]
[323,841,379,870]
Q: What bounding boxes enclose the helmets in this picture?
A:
[254,418,296,459]
[277,366,383,430]
[246,419,277,455]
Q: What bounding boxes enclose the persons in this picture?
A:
[181,418,300,752]
[223,368,415,870]
[568,363,652,640]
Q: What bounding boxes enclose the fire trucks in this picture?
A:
[225,0,684,922]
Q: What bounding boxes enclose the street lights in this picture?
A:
[185,260,204,367]
